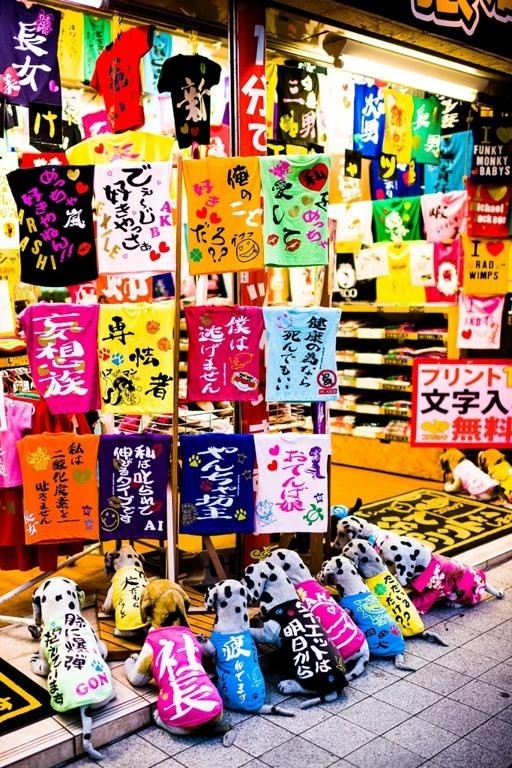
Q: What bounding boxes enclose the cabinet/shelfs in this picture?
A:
[327,300,460,487]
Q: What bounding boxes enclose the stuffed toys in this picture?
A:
[266,548,371,680]
[438,447,500,502]
[476,447,512,505]
[197,578,298,719]
[121,579,232,742]
[240,559,351,709]
[29,575,116,759]
[314,495,505,671]
[100,543,149,638]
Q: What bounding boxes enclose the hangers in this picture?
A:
[0,364,43,418]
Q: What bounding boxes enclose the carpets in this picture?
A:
[0,655,59,735]
[351,487,511,555]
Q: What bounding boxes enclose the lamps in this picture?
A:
[319,29,483,105]
